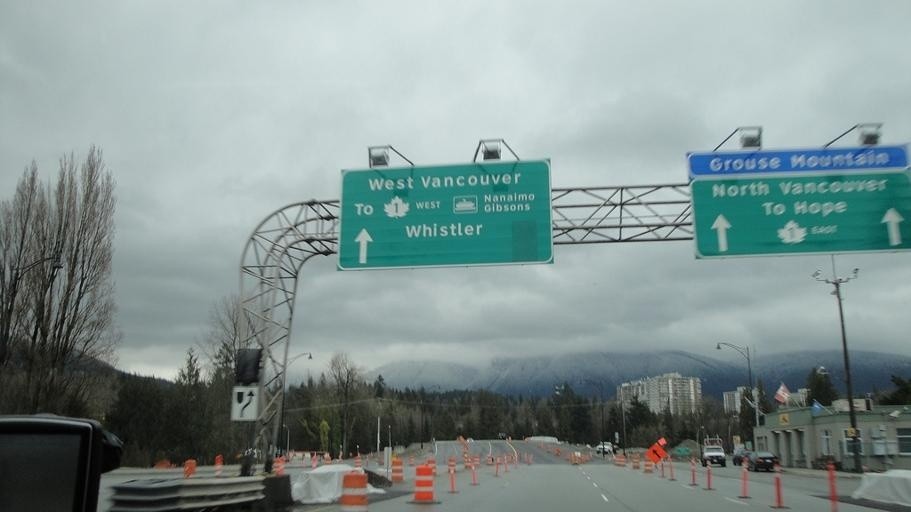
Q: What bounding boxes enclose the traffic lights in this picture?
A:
[0,408,132,512]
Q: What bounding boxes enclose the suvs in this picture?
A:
[699,445,727,469]
[595,441,612,455]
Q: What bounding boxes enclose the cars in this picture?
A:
[732,448,752,466]
[744,449,779,473]
[465,437,474,442]
[496,432,506,441]
[613,445,620,453]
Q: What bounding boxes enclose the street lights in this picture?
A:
[696,425,704,457]
[281,424,290,452]
[2,254,63,360]
[811,267,863,475]
[715,340,760,426]
[419,383,440,449]
[285,351,314,372]
[579,379,607,442]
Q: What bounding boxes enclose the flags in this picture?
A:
[775,383,791,404]
[811,401,821,417]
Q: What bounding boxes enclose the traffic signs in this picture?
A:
[688,167,911,260]
[334,156,553,274]
[681,142,911,174]
